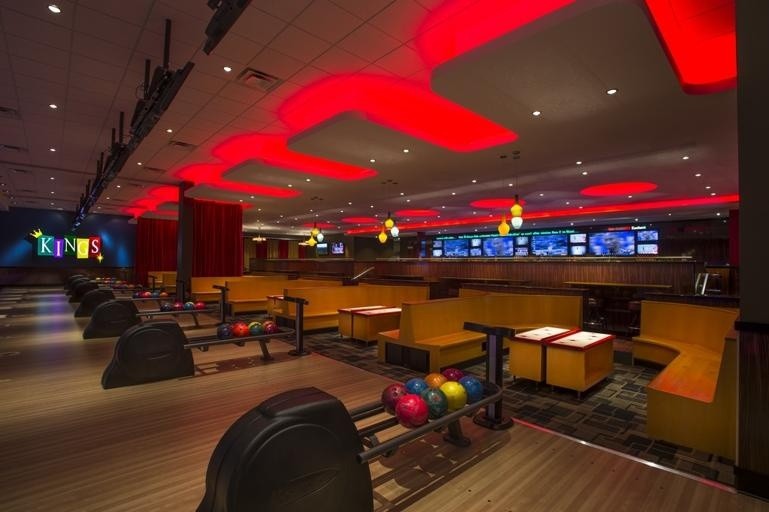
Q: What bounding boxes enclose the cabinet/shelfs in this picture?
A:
[509,323,617,400]
[266,294,284,317]
[337,304,402,347]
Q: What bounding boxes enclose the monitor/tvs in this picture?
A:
[636,244,660,255]
[420,240,426,257]
[570,245,587,256]
[608,225,631,230]
[569,234,587,243]
[431,236,529,258]
[202,0,253,56]
[316,242,329,255]
[530,235,569,256]
[636,229,661,243]
[331,242,345,255]
[632,226,648,231]
[72,60,195,232]
[588,232,636,256]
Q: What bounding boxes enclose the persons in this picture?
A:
[600,233,632,256]
[491,238,509,256]
[547,242,553,256]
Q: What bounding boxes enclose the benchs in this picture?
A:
[145,270,177,288]
[270,282,431,332]
[218,277,343,321]
[632,299,741,461]
[159,271,177,294]
[191,274,244,303]
[377,287,584,373]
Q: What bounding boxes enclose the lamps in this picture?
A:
[498,149,523,236]
[378,177,399,243]
[308,196,325,247]
